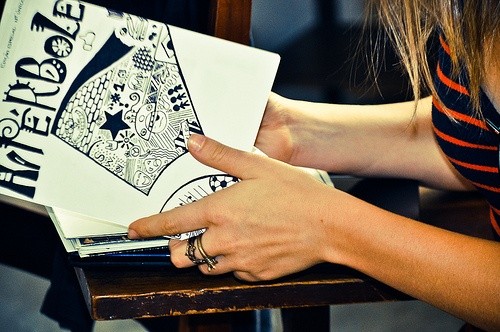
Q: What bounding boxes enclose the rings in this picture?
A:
[197,234,218,273]
[184,233,206,265]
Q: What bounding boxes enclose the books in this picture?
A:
[0,0,341,266]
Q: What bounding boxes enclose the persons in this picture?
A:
[128,1,500,331]
